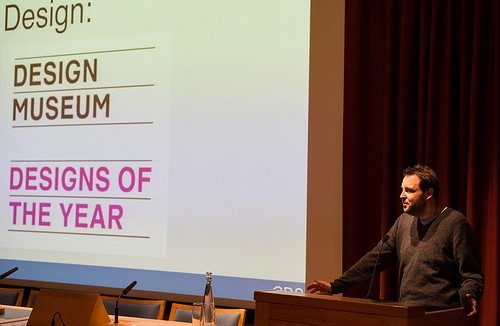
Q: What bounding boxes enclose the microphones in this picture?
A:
[0,266,19,281]
[363,234,391,299]
[114,280,137,323]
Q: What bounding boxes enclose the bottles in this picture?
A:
[201,272,216,325]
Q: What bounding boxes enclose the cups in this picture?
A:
[192,302,204,326]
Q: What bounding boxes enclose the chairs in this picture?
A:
[101,295,165,321]
[168,303,246,326]
[0,287,39,309]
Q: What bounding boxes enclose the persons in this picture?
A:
[306,163,485,317]
